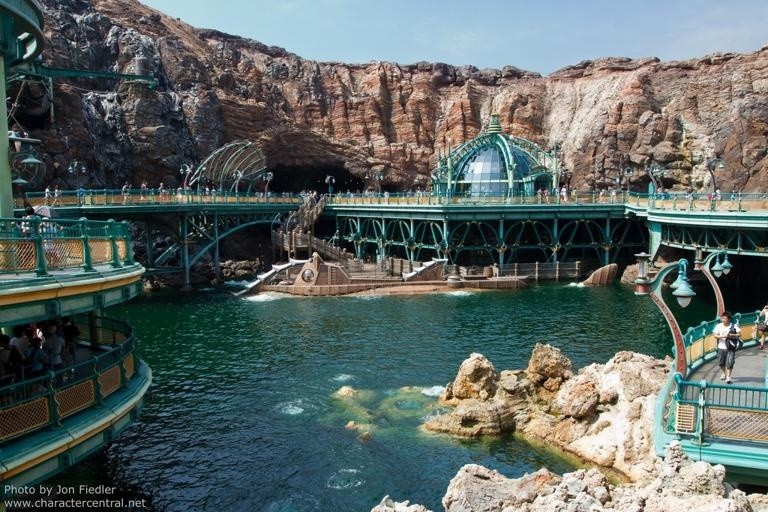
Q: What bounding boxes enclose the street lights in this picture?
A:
[644,156,665,194]
[67,161,88,203]
[633,251,698,428]
[325,176,335,197]
[438,138,452,189]
[624,167,633,192]
[179,160,196,188]
[693,245,733,318]
[545,140,565,188]
[365,170,385,198]
[705,159,724,193]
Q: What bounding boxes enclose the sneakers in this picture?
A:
[720,374,731,383]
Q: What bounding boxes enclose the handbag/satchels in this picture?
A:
[760,324,768,331]
[726,328,743,350]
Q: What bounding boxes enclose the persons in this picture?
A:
[757,305,768,350]
[537,185,737,211]
[713,311,742,383]
[21,205,64,267]
[0,317,80,405]
[44,180,390,206]
